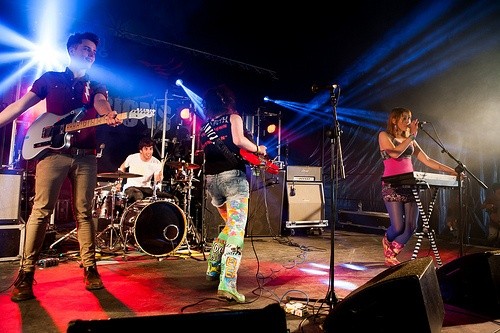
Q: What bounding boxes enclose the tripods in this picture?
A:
[182,167,214,261]
[94,177,128,259]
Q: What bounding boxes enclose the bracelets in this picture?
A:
[255,146,260,154]
[409,135,415,140]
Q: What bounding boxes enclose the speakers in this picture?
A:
[244,166,285,236]
[205,189,226,244]
[321,256,445,333]
[436,250,500,319]
[66,302,288,333]
[286,182,325,222]
[0,169,24,225]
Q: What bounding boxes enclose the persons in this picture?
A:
[199,86,267,301]
[482,183,500,239]
[118,139,163,200]
[379,108,467,268]
[0,32,123,302]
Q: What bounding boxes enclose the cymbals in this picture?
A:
[166,162,200,169]
[97,172,144,178]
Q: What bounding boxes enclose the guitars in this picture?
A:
[21,107,157,162]
[238,129,279,175]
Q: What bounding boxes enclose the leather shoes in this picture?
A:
[49,148,97,156]
[7,271,34,301]
[83,265,104,290]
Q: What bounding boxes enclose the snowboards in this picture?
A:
[380,171,463,270]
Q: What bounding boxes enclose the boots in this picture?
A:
[216,254,246,303]
[382,233,391,255]
[206,242,225,282]
[384,240,406,268]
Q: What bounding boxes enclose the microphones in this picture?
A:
[312,83,339,93]
[408,121,429,128]
[290,185,295,196]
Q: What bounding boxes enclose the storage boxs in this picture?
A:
[0,217,26,261]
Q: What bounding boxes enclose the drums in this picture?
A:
[94,194,128,221]
[119,199,188,259]
